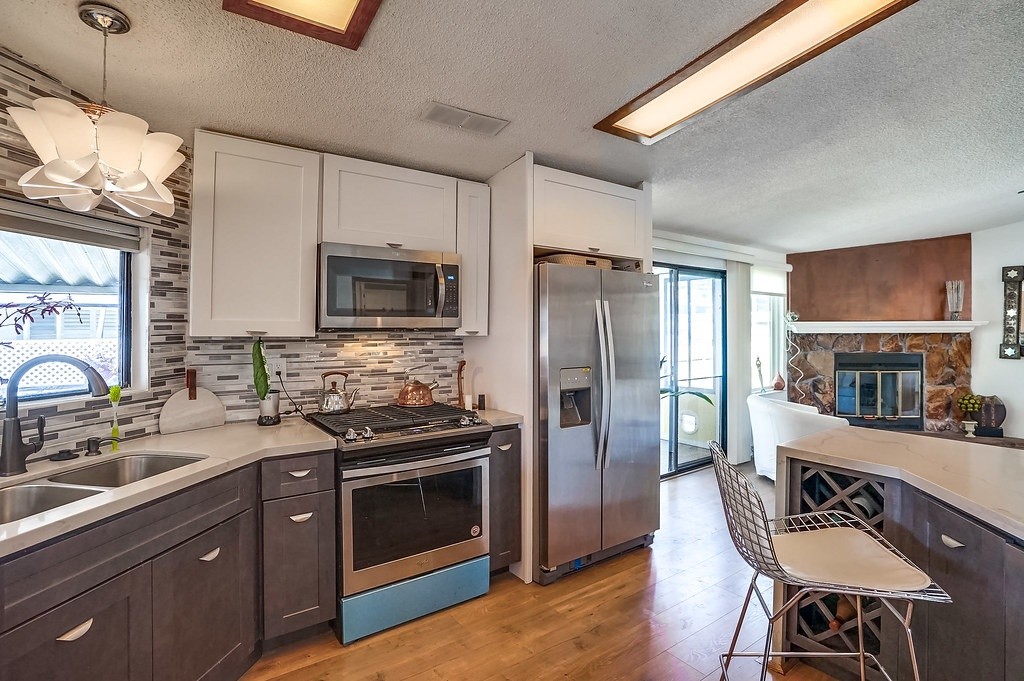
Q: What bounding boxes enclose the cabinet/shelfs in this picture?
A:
[835,352,923,431]
[488,428,521,571]
[0,460,262,681]
[319,150,491,337]
[190,127,319,336]
[532,163,643,261]
[262,449,337,653]
[784,457,1024,681]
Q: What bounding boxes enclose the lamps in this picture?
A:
[7,3,186,217]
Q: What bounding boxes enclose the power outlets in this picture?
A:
[270,358,286,381]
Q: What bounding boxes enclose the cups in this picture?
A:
[258,390,282,426]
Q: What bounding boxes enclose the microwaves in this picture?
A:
[317,241,463,339]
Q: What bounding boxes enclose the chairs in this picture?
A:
[706,438,954,681]
[747,389,848,483]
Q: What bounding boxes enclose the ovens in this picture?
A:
[334,444,490,598]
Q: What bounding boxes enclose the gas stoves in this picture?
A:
[307,400,493,456]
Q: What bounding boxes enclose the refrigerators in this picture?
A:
[533,261,661,584]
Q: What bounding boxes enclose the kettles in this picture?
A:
[318,371,360,414]
[395,363,440,408]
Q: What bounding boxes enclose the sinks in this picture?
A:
[44,450,212,489]
[0,485,105,527]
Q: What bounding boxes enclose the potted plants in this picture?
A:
[957,394,981,438]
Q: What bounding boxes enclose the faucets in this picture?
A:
[84,437,122,457]
[0,354,108,477]
